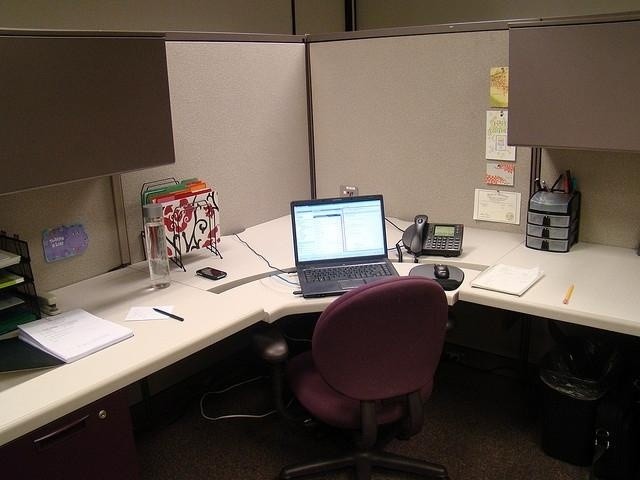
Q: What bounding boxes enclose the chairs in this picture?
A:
[278,273,452,478]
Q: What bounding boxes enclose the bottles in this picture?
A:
[143,203,171,288]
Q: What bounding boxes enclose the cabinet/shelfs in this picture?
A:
[0,26,178,197]
[506,13,640,158]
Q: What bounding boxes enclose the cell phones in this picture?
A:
[196,267,228,280]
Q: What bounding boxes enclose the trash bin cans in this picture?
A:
[539,368,604,466]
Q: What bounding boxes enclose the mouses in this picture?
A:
[434,260,451,282]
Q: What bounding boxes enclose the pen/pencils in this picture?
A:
[564,170,576,192]
[536,174,564,192]
[153,308,184,322]
[564,285,574,304]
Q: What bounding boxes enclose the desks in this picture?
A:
[0,264,264,479]
[128,215,640,339]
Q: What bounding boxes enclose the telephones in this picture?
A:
[402,215,463,256]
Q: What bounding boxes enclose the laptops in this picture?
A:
[289,195,404,296]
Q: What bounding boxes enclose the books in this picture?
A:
[0,250,136,366]
